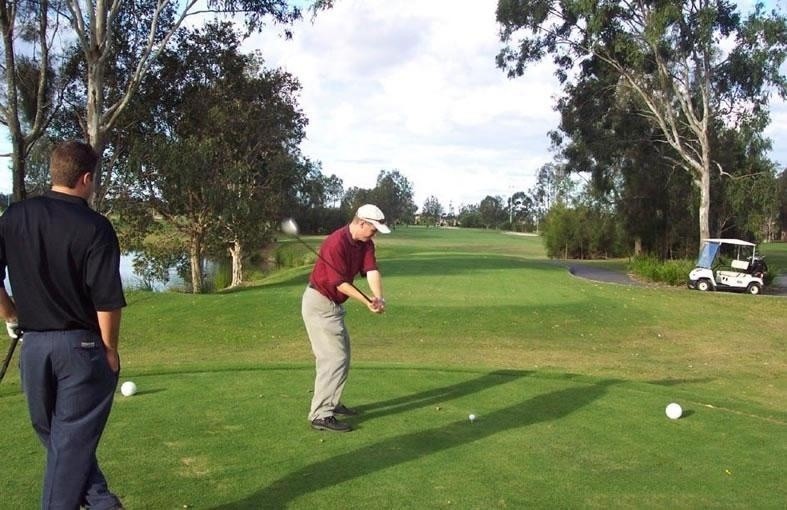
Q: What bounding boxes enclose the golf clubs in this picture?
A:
[280,217,373,303]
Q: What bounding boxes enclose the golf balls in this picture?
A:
[121,382,136,396]
[468,414,476,420]
[665,403,682,419]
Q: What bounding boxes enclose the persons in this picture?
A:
[0,137,127,509]
[298,201,393,435]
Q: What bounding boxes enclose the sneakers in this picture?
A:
[312,417,353,432]
[333,402,358,417]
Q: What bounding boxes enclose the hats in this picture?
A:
[355,204,391,234]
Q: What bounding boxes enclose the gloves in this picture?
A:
[5,318,24,342]
